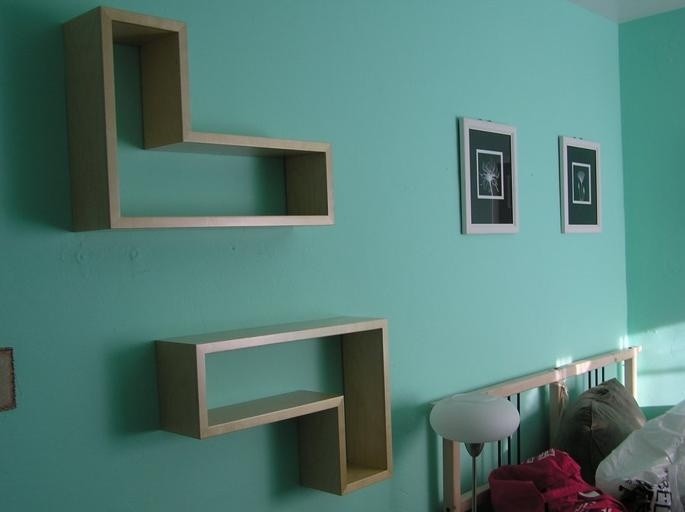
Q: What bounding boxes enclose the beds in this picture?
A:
[424,346,642,511]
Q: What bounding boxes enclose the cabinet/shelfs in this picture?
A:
[59,7,335,230]
[150,316,392,495]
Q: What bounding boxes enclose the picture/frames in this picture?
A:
[559,134,601,236]
[459,115,519,234]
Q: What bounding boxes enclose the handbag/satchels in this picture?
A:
[488,447,626,512]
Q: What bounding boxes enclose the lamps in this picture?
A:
[427,394,523,510]
